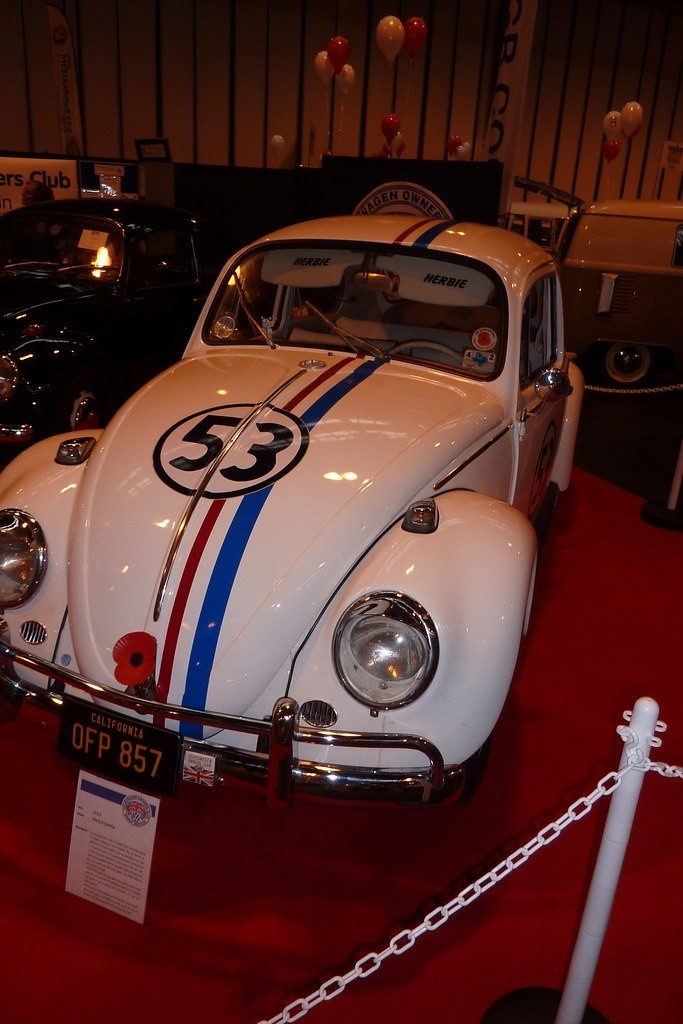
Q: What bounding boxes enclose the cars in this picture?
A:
[545,197,683,391]
[0,210,585,808]
[0,188,217,469]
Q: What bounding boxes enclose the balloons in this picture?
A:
[461,141,472,155]
[395,141,406,156]
[381,113,400,148]
[372,150,390,159]
[321,149,333,160]
[619,100,645,140]
[383,131,404,149]
[445,136,457,158]
[452,145,466,160]
[312,49,339,84]
[335,63,356,98]
[271,133,285,156]
[326,34,351,76]
[401,16,428,60]
[602,138,622,163]
[602,111,625,139]
[375,15,404,66]
[377,133,392,152]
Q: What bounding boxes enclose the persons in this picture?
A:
[20,178,56,208]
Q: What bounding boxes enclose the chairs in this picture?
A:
[289,328,398,359]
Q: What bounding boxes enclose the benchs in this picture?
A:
[331,318,500,374]
